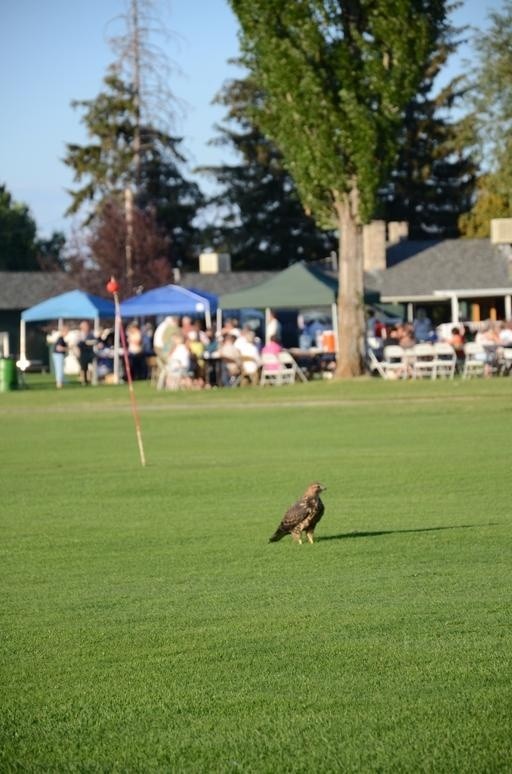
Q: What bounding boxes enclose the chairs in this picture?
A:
[89,319,512,387]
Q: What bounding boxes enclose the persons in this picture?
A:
[366,307,512,381]
[39,306,283,391]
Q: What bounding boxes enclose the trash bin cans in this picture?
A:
[0,356,15,393]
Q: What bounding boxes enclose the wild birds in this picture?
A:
[267,482,325,544]
